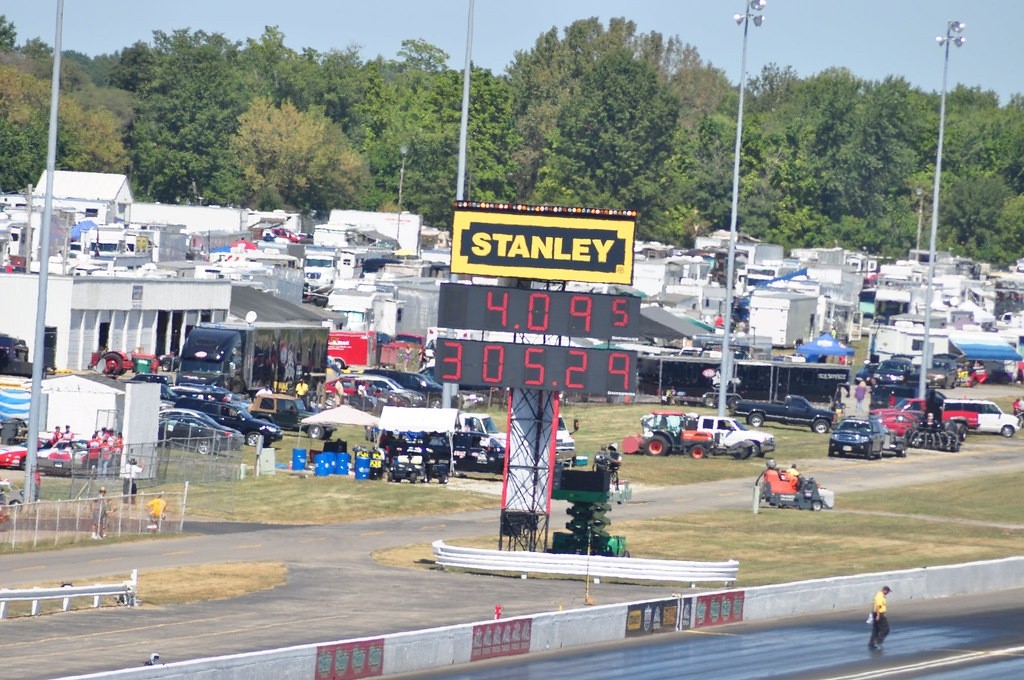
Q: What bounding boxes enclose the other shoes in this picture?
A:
[92,535,100,539]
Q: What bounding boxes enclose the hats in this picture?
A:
[883,586,892,592]
[99,487,106,491]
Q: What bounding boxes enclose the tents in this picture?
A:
[798,333,855,366]
[298,404,380,455]
[379,404,465,476]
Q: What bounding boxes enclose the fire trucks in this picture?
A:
[327,329,376,369]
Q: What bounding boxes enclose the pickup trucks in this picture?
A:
[641,416,776,458]
[870,383,980,441]
[737,395,836,433]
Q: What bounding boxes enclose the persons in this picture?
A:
[787,464,801,479]
[869,586,892,649]
[254,377,381,410]
[665,386,676,404]
[382,433,408,464]
[1013,361,1024,427]
[33,424,168,538]
[592,443,622,491]
[855,378,888,414]
[716,316,724,328]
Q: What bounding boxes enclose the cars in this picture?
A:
[854,353,962,388]
[124,370,486,456]
[0,481,24,523]
[0,434,91,476]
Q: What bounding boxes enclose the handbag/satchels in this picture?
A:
[866,614,873,624]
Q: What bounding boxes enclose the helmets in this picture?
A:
[767,460,776,468]
[601,445,606,451]
[130,459,137,465]
[791,464,796,468]
[65,425,69,428]
[608,444,617,449]
[93,427,122,443]
[55,426,58,428]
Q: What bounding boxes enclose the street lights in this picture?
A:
[918,21,968,400]
[716,0,767,418]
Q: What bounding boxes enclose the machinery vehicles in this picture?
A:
[100,345,159,379]
[621,410,713,458]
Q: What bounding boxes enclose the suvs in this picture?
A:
[943,397,1021,439]
[827,416,885,459]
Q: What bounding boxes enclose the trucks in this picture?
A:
[378,406,506,448]
[554,414,579,453]
[0,333,29,375]
[176,315,329,395]
[302,244,343,289]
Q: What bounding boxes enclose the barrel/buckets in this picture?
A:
[355,445,386,479]
[313,439,351,478]
[292,449,306,469]
